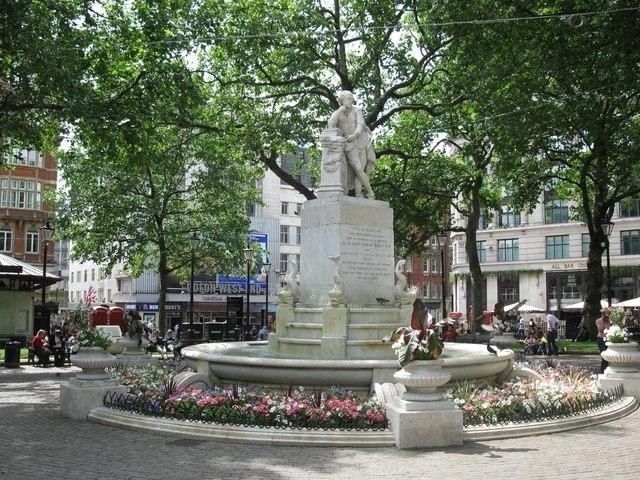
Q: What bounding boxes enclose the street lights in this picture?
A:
[262,254,271,331]
[189,230,201,339]
[39,218,55,329]
[435,227,447,318]
[601,213,615,309]
[244,243,252,340]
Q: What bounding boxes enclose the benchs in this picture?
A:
[25,339,71,368]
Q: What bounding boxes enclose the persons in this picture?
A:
[234,325,240,342]
[328,90,377,200]
[544,310,560,356]
[258,326,268,339]
[152,329,167,362]
[32,330,49,367]
[165,330,183,358]
[596,309,614,373]
[536,332,547,357]
[252,325,256,339]
[527,333,537,353]
[49,325,66,368]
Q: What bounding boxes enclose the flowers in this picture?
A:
[601,305,638,344]
[75,329,114,350]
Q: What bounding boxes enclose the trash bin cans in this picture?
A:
[5,341,21,367]
